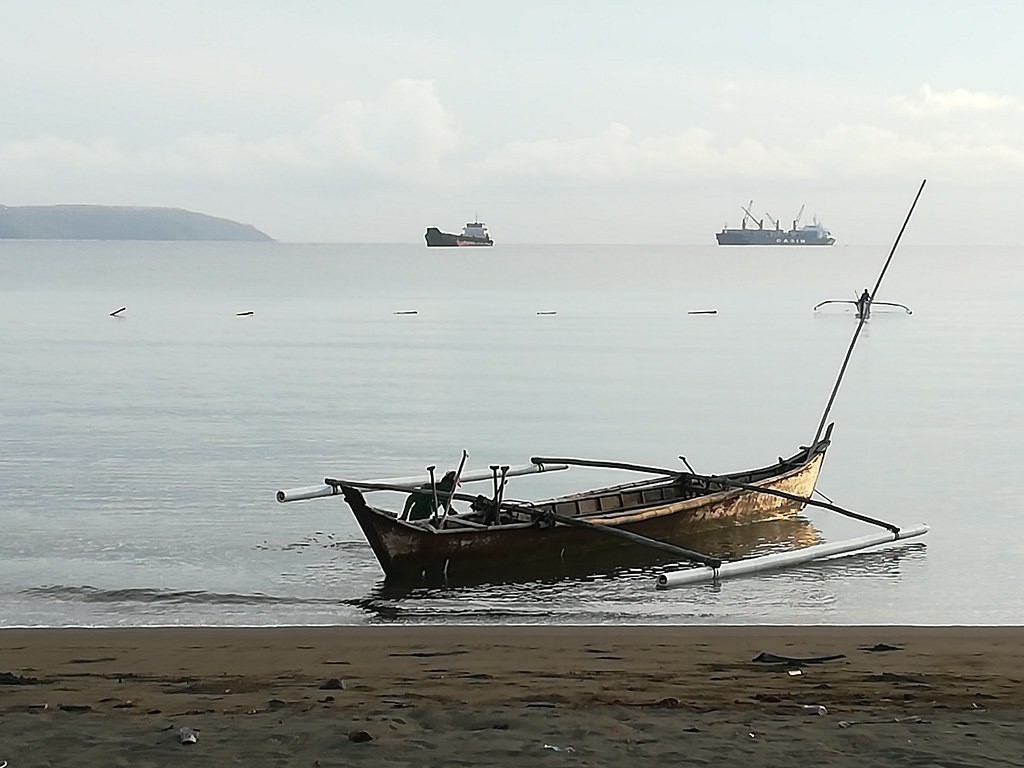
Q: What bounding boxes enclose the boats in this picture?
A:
[855,297,871,318]
[715,201,836,245]
[425,214,493,246]
[278,423,855,577]
